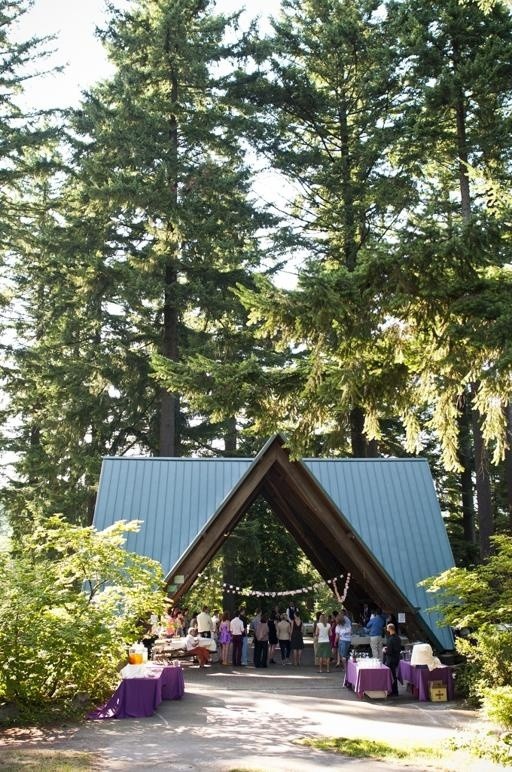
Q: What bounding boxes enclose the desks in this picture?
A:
[344,628,458,705]
[85,630,218,721]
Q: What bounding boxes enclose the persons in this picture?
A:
[382,624,401,696]
[386,614,398,636]
[367,607,384,660]
[169,606,351,675]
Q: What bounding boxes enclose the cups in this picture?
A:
[349,652,383,669]
